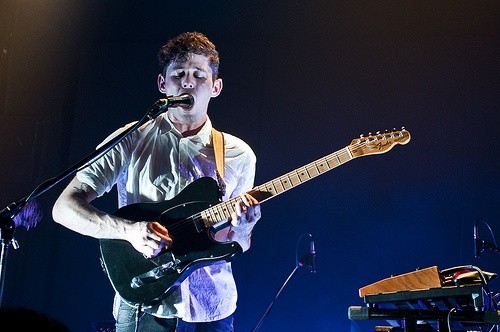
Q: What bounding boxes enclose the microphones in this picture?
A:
[305,235,316,273]
[157,93,193,109]
[472,220,482,259]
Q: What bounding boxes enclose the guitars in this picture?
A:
[101,125,412,306]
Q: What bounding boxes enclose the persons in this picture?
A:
[51,32,262,331]
